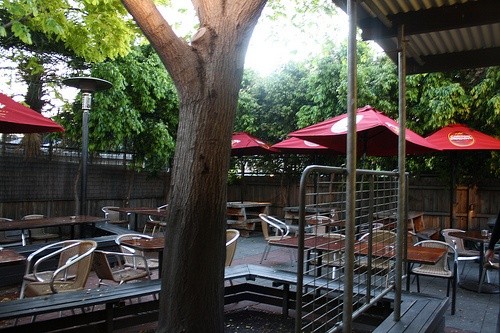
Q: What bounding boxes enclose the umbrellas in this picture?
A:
[424,123,500,228]
[288,104,442,241]
[271,137,346,208]
[230,133,282,204]
[0,93,65,133]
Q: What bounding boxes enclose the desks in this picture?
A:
[269,237,446,292]
[120,237,164,279]
[372,211,427,245]
[0,249,26,266]
[0,215,109,249]
[449,232,500,293]
[108,207,166,232]
[283,207,340,236]
[226,201,271,241]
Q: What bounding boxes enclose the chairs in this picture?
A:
[0,204,239,324]
[258,213,500,314]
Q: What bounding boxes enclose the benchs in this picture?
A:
[420,226,440,238]
[0,222,138,290]
[0,262,442,333]
[228,216,275,225]
